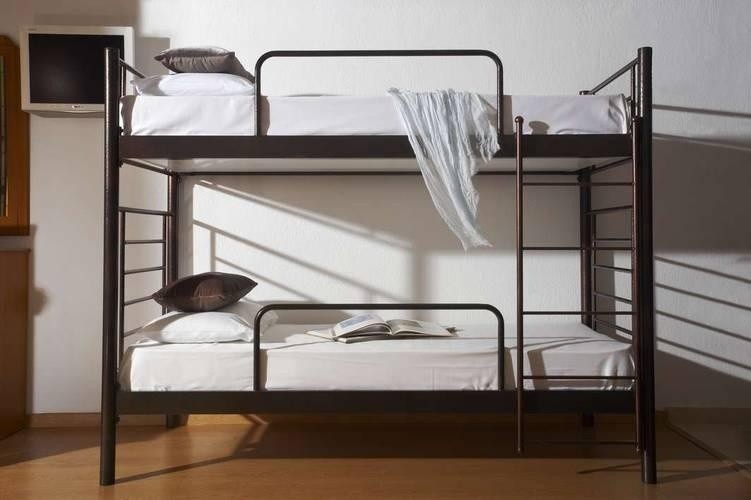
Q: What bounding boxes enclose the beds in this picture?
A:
[98,47,655,487]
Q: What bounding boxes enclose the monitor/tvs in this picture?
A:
[19,25,135,112]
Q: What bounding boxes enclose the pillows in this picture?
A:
[152,271,258,313]
[139,303,278,344]
[130,73,255,96]
[155,48,255,84]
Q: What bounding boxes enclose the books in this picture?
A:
[328,312,454,340]
[304,327,457,344]
